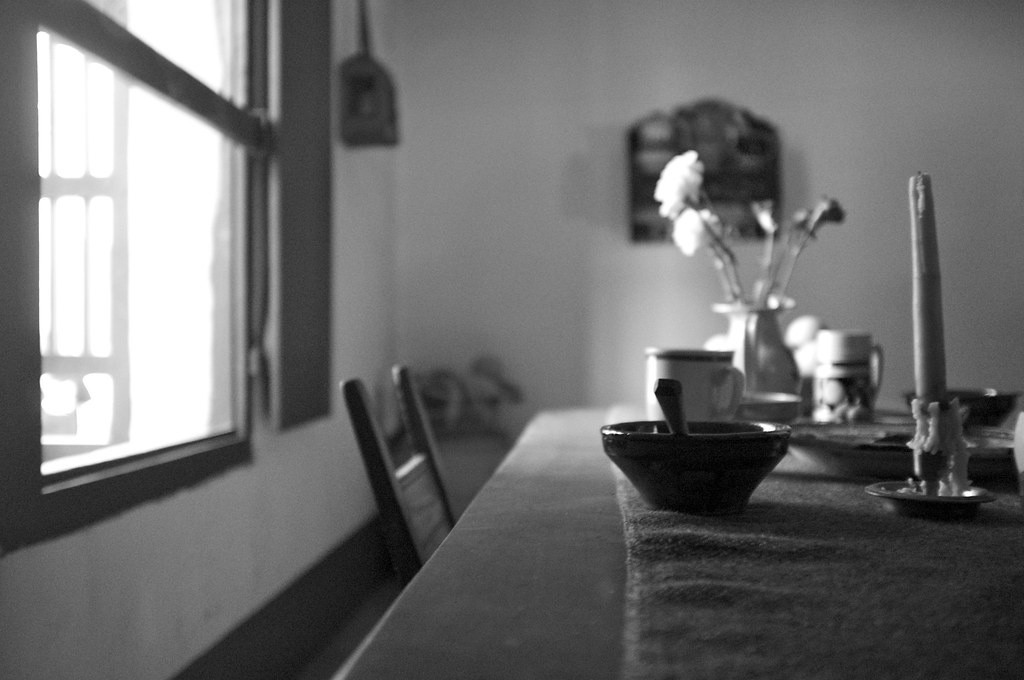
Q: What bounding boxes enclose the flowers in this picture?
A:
[654,150,844,304]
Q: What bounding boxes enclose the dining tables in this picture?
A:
[333,408,1023,680]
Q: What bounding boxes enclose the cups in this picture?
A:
[647,345,747,424]
[810,327,885,422]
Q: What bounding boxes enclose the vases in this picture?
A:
[713,300,801,396]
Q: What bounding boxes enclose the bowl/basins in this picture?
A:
[740,392,802,427]
[904,390,1019,430]
[597,417,791,512]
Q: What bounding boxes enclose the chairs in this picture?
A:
[341,365,456,592]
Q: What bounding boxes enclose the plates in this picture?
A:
[865,481,994,519]
[787,425,1015,484]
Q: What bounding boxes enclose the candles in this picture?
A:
[909,170,959,452]
